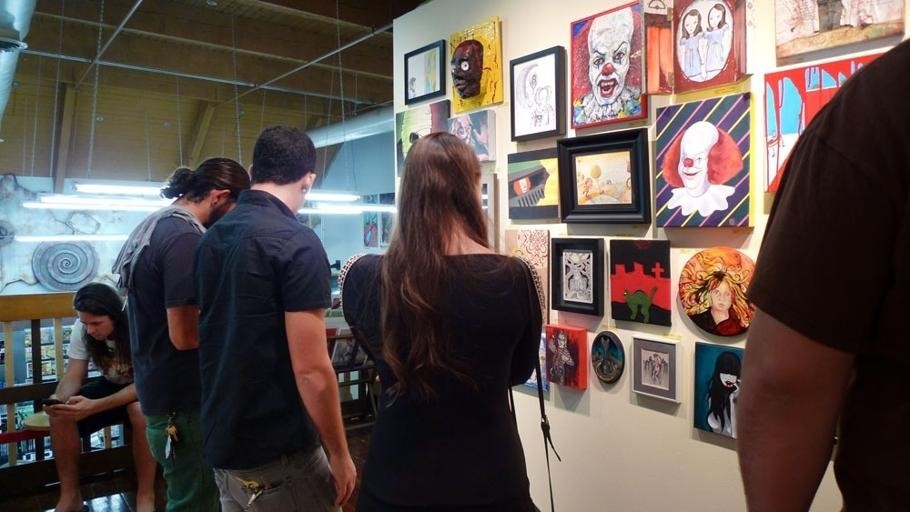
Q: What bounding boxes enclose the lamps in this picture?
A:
[13,0,182,243]
[296,1,395,215]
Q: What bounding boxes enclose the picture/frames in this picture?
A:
[509,45,568,143]
[550,237,605,320]
[404,37,448,106]
[626,332,682,407]
[556,126,653,226]
[477,171,498,251]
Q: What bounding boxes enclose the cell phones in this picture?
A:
[41,398,65,406]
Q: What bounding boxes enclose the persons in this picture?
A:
[342,338,360,367]
[573,7,641,125]
[195,124,359,511]
[43,279,162,512]
[449,40,484,99]
[677,9,704,83]
[702,4,732,80]
[337,130,545,511]
[678,247,762,336]
[705,351,743,440]
[735,38,909,511]
[117,156,224,512]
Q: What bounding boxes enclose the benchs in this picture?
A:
[24,405,134,490]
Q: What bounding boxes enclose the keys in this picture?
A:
[164,422,179,466]
[234,475,259,492]
[247,487,264,506]
